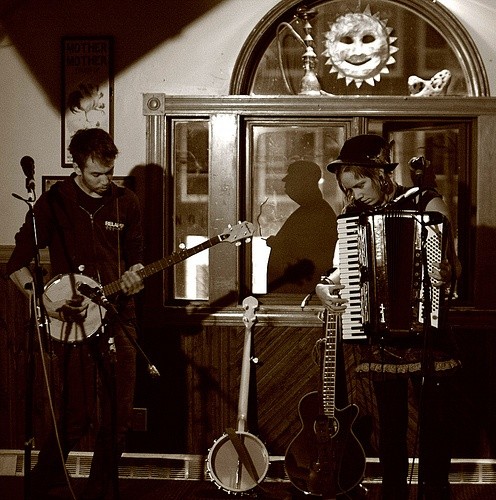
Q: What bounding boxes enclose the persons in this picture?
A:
[315,135,463,500]
[6,128,145,500]
[264,161,336,294]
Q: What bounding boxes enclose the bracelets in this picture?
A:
[317,276,335,285]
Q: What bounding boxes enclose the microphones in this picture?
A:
[75,281,111,307]
[20,156,36,190]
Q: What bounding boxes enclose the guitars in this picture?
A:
[284,305,366,496]
[36,219,257,343]
[206,294,269,494]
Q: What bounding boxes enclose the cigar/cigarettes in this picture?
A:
[261,238,267,240]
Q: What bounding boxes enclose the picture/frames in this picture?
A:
[60,34,114,165]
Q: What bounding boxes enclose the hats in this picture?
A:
[326,135,399,172]
